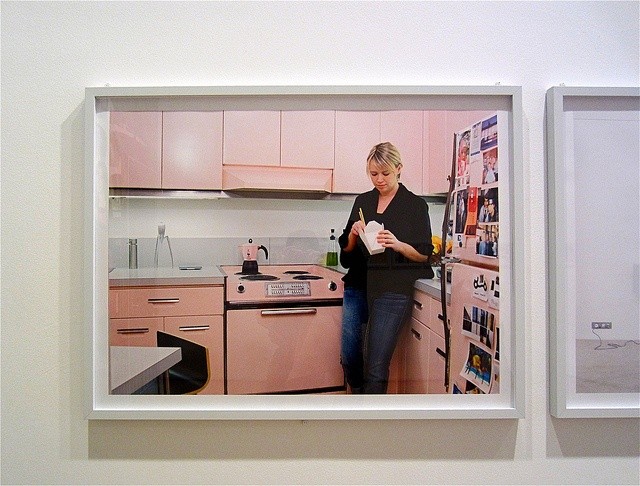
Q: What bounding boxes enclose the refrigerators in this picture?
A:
[448,111,513,394]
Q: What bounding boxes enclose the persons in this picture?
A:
[474,349,489,386]
[466,346,474,374]
[478,196,488,220]
[477,233,498,257]
[457,135,470,185]
[456,235,463,247]
[458,192,465,216]
[338,142,434,394]
[487,196,496,220]
[483,152,497,183]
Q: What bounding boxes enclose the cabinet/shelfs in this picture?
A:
[109,111,163,187]
[422,111,491,197]
[162,110,225,189]
[332,112,423,194]
[108,285,224,395]
[396,290,444,393]
[223,108,334,170]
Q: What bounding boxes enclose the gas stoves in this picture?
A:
[221,263,347,304]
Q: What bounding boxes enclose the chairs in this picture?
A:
[135,330,212,396]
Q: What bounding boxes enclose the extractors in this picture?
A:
[221,165,332,200]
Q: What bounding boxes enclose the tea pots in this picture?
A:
[240,238,268,273]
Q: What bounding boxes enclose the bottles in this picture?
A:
[325,228,339,267]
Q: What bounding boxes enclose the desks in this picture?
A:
[109,345,182,395]
